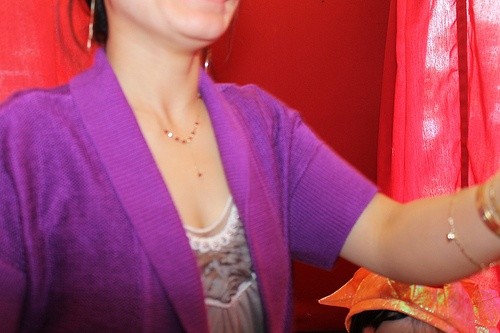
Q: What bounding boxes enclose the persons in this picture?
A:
[0,0,500,333]
[363,312,443,333]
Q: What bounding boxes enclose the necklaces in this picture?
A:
[139,103,203,178]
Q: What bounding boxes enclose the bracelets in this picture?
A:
[443,173,500,269]
